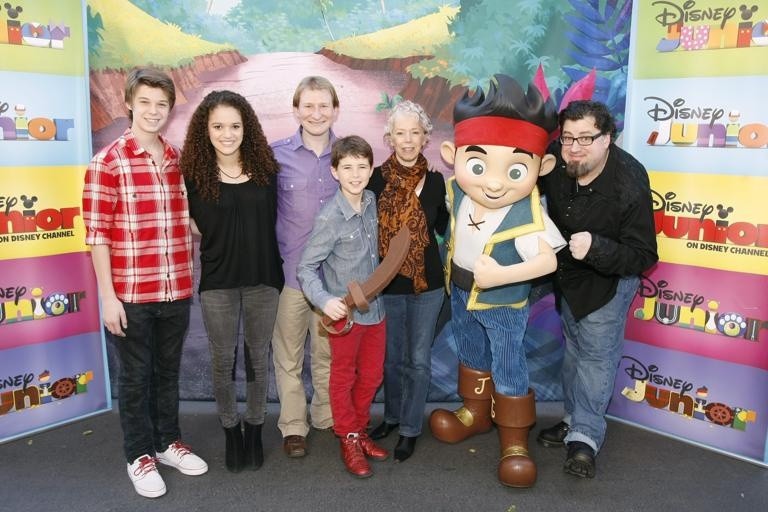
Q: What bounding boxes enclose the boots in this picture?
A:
[222,420,246,474]
[243,420,264,472]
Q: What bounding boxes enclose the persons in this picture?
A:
[536,100,659,479]
[295,136,389,479]
[262,75,344,458]
[429,73,567,489]
[368,101,453,464]
[82,69,208,500]
[178,90,285,473]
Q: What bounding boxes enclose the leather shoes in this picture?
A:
[369,422,400,442]
[394,435,417,463]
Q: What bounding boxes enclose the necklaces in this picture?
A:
[219,168,243,179]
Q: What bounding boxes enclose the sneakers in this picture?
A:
[563,441,596,479]
[156,439,208,476]
[284,436,308,459]
[314,419,374,432]
[536,421,570,449]
[358,432,390,463]
[126,453,167,499]
[339,437,374,480]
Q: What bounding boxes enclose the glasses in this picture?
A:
[559,132,603,146]
